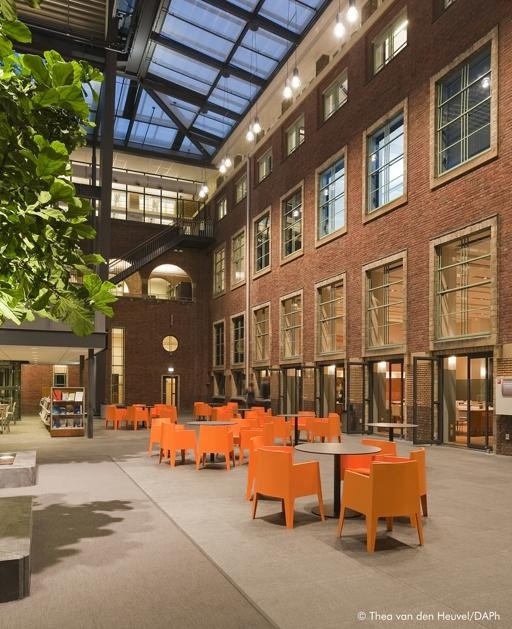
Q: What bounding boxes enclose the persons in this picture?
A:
[245,382,254,408]
[261,376,271,408]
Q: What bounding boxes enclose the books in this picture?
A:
[40,389,83,428]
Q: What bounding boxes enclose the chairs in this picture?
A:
[0,402,16,435]
[456,400,493,437]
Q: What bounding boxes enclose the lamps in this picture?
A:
[218,69,233,177]
[245,19,262,144]
[282,1,300,104]
[333,0,361,41]
[199,112,209,201]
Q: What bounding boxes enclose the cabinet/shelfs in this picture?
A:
[39,387,86,437]
